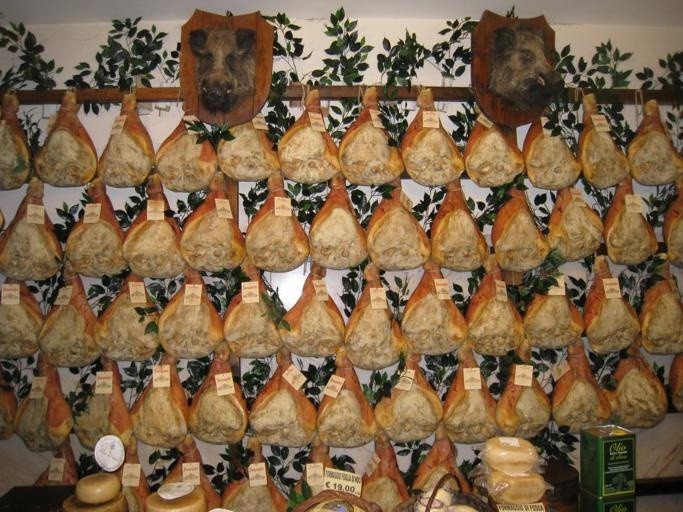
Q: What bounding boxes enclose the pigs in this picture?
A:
[488,25,565,113]
[187,27,258,113]
